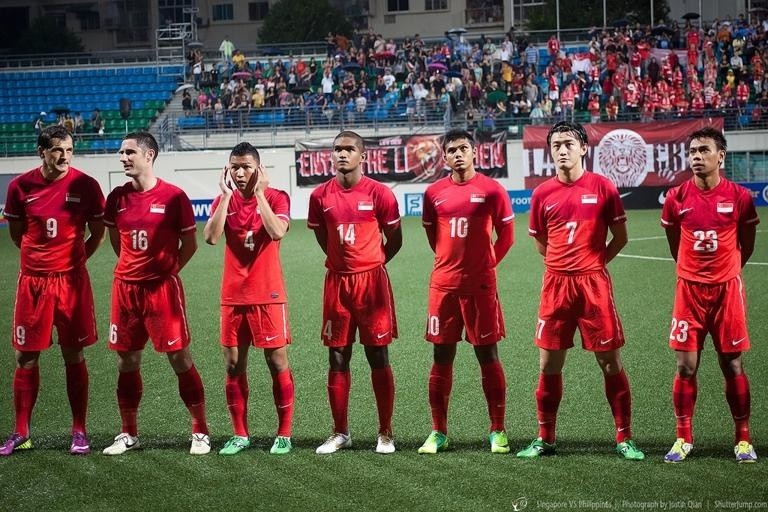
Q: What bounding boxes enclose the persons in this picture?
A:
[102,130,212,459]
[417,129,516,458]
[657,127,761,466]
[27,106,108,142]
[305,129,406,458]
[515,121,645,462]
[173,14,767,134]
[3,123,103,461]
[203,141,293,459]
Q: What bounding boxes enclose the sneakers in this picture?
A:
[516,438,556,458]
[1,434,33,455]
[271,437,291,454]
[316,432,352,455]
[375,432,395,453]
[70,433,90,455]
[104,433,140,455]
[665,435,695,463]
[419,429,448,453]
[616,439,645,460]
[489,431,511,453]
[734,440,758,462]
[220,436,251,456]
[189,433,211,455]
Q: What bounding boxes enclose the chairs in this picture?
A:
[1,21,767,158]
